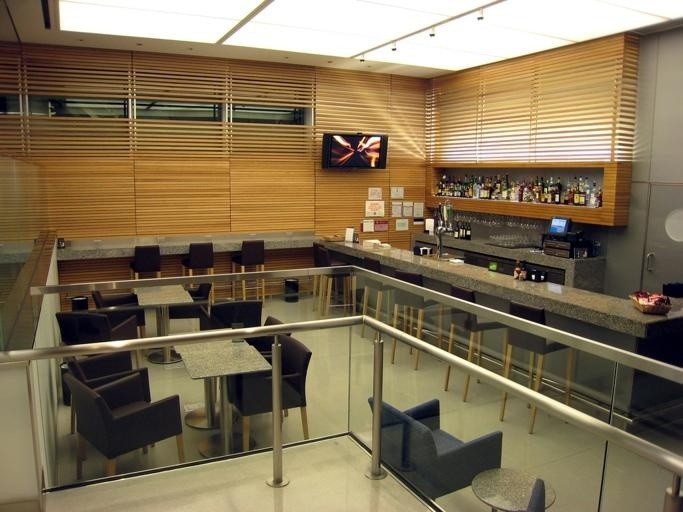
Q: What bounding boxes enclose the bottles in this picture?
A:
[434,169,601,207]
[433,200,472,240]
[514,257,549,281]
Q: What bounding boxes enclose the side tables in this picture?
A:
[471,468,557,511]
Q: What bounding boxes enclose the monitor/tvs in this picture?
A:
[322,132,389,170]
[548,216,570,238]
